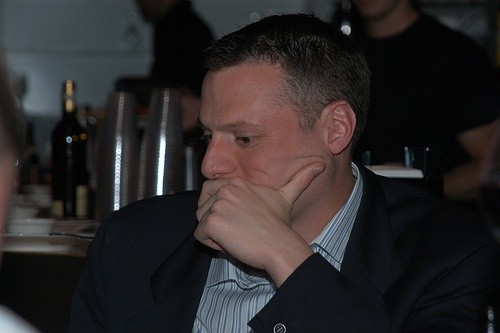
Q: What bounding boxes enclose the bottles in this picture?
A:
[25,79,96,220]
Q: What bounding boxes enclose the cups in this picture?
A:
[404,146,429,172]
[95,92,199,233]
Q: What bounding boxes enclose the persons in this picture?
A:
[334,0,500,206]
[114,0,216,130]
[67,13,500,333]
[0,46,43,333]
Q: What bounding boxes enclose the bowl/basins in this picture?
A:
[4,185,54,234]
